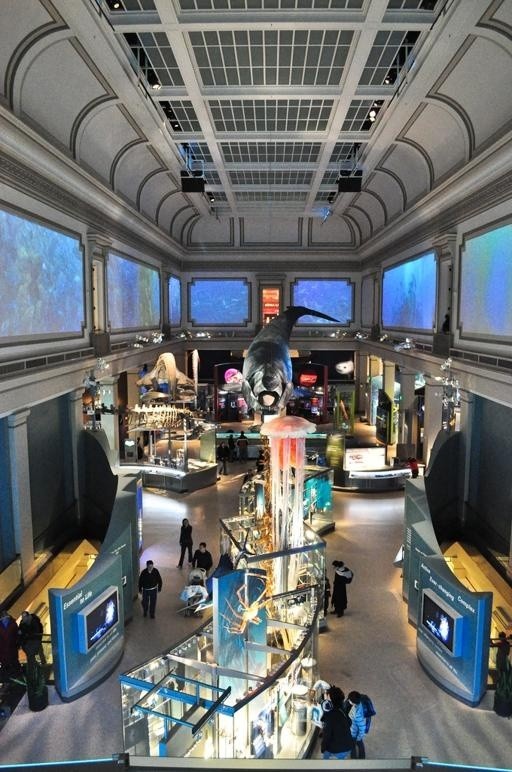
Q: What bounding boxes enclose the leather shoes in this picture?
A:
[330,611,343,617]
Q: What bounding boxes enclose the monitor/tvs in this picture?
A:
[420,588,464,658]
[76,584,122,656]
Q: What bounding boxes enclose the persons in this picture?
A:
[175,518,194,568]
[255,449,265,472]
[227,434,236,463]
[327,559,354,618]
[191,542,213,578]
[407,456,418,478]
[488,631,511,672]
[236,430,249,465]
[344,689,367,759]
[12,611,48,665]
[321,567,331,618]
[136,437,144,460]
[1,608,20,667]
[316,684,355,760]
[440,314,450,333]
[138,560,163,620]
[216,442,229,475]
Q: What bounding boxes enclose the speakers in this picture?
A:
[338,178,362,193]
[180,177,206,193]
